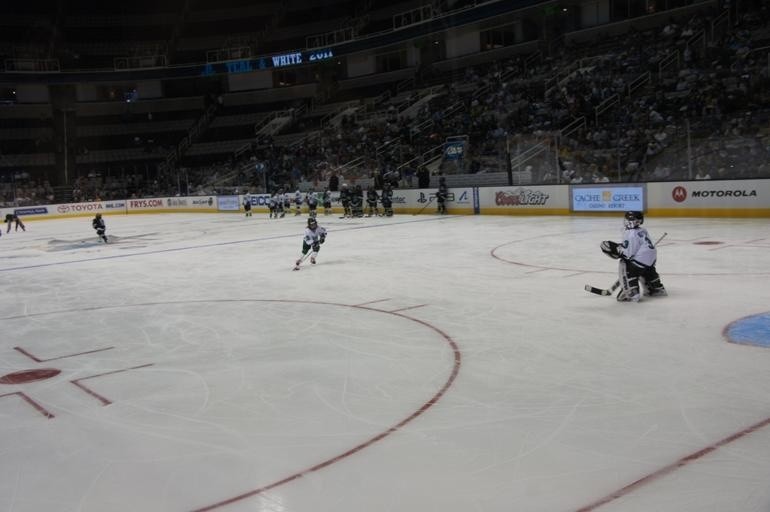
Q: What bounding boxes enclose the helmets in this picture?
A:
[624,211,643,229]
[307,218,317,230]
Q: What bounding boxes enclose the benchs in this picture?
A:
[1,39,770,213]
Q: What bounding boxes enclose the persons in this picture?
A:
[93,213,109,244]
[296,218,327,270]
[0,1,768,220]
[5,214,25,232]
[602,211,664,298]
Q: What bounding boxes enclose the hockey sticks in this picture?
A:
[586,232,667,296]
[292,249,314,271]
[413,196,436,217]
